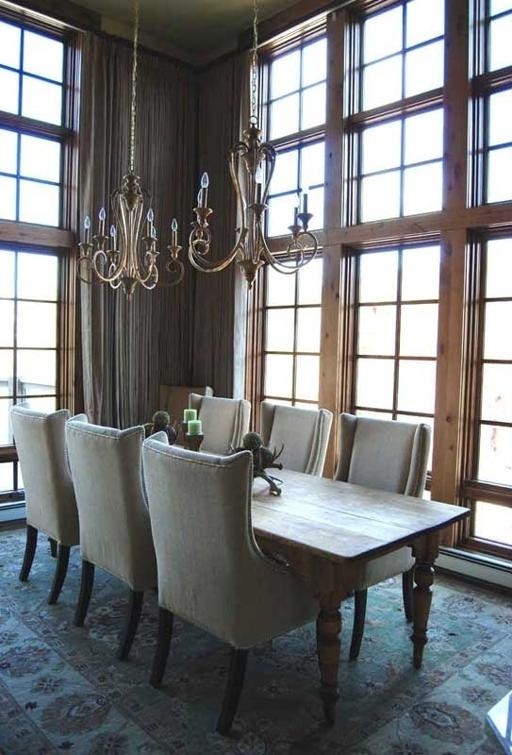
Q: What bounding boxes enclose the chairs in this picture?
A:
[10,402,79,605]
[187,392,251,456]
[259,401,333,477]
[141,431,322,738]
[333,412,433,661]
[65,412,158,660]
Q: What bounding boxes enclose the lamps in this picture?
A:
[187,0,319,290]
[76,0,186,302]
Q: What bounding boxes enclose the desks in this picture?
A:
[252,468,472,727]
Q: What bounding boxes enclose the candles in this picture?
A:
[188,420,201,435]
[184,409,198,424]
